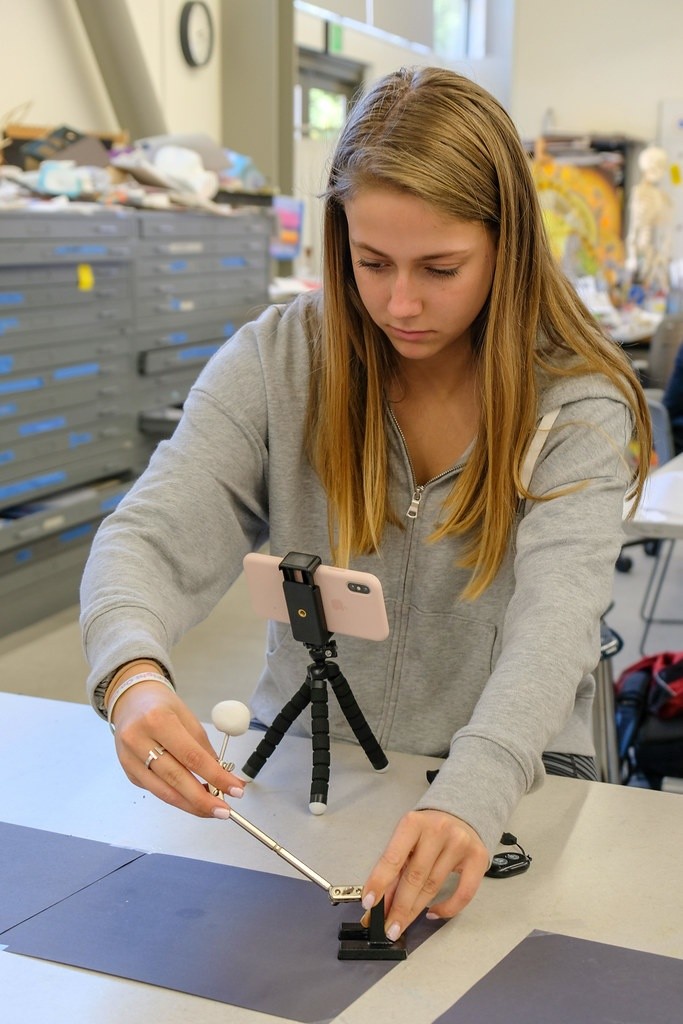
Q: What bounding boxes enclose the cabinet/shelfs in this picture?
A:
[1,212,279,633]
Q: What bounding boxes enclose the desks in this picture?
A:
[621,450,683,657]
[0,693,682,1024]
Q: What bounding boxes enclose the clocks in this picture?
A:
[181,1,213,66]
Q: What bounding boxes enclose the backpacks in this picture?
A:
[613,652,683,790]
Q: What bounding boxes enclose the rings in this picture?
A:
[144,746,166,770]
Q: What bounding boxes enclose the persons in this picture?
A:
[77,58,655,943]
[650,314,683,457]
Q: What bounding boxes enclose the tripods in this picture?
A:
[238,658,391,816]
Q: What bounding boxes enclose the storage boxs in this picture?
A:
[2,126,130,170]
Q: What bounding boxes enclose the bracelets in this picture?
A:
[106,671,176,736]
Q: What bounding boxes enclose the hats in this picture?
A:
[6,159,112,204]
[200,146,276,200]
[110,144,219,199]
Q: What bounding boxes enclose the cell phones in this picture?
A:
[242,552,390,640]
